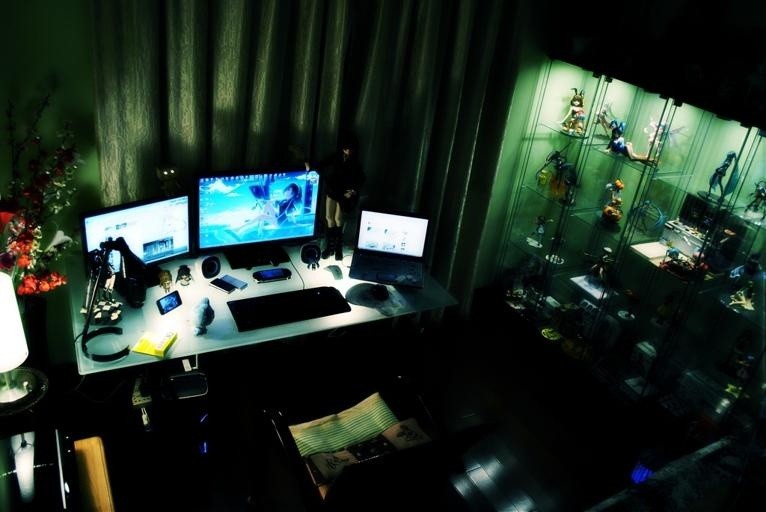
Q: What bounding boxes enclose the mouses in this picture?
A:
[373,285,389,301]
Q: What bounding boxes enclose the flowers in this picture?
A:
[2,77,86,296]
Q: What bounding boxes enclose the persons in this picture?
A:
[492,84,765,453]
[174,264,193,287]
[158,268,174,293]
[665,150,765,312]
[303,132,360,261]
[542,92,657,235]
[506,210,765,452]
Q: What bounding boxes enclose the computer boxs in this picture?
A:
[155,364,208,457]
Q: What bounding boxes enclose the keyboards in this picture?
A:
[228,287,351,332]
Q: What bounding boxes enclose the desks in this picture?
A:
[67,234,457,420]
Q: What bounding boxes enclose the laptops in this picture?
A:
[350,206,431,289]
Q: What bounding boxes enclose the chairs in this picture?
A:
[247,388,452,512]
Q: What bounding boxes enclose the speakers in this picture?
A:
[195,256,220,280]
[299,240,321,267]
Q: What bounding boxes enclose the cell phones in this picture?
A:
[253,267,292,283]
[157,291,182,315]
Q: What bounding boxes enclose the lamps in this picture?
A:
[1,271,35,401]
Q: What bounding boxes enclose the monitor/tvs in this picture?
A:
[198,168,322,269]
[79,194,191,286]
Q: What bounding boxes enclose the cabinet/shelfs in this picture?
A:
[484,53,766,439]
